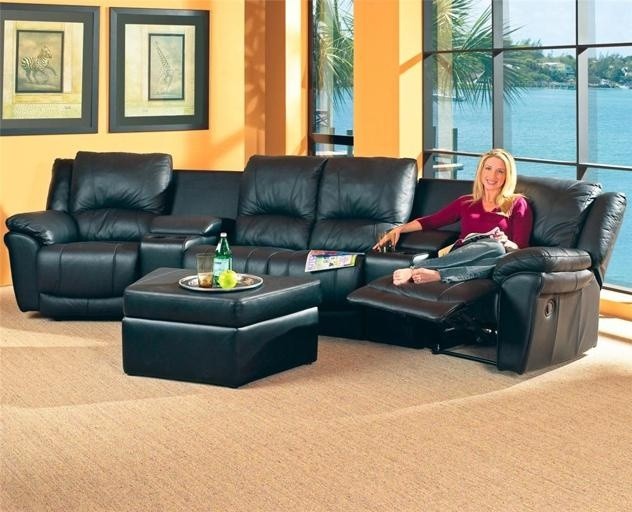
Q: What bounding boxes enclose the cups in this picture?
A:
[196,253,212,286]
[377,232,396,253]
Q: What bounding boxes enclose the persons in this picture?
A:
[371,147,536,286]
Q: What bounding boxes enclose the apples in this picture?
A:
[216,270,238,289]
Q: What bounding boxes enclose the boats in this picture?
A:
[432,90,467,103]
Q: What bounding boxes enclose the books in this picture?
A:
[304,248,365,273]
[438,226,499,259]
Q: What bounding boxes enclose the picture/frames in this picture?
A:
[1,2,101,139]
[108,7,211,135]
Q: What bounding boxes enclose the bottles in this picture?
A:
[211,231,232,286]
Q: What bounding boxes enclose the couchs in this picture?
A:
[4,151,627,374]
